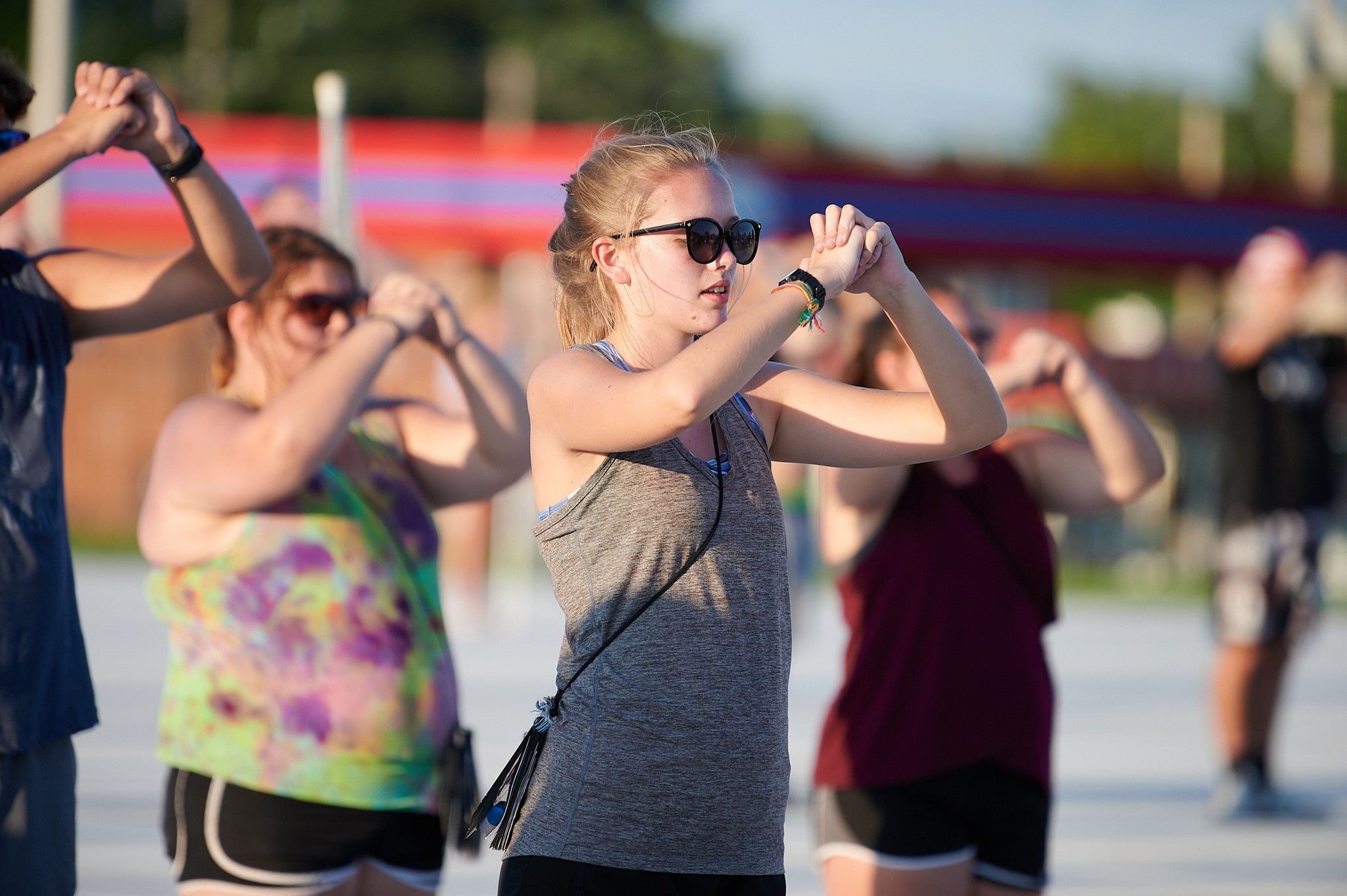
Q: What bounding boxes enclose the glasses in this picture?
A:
[589,218,762,266]
[0,129,30,154]
[296,288,368,327]
[967,321,997,350]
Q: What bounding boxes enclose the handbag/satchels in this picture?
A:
[447,723,482,854]
[461,708,549,855]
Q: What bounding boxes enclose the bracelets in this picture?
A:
[445,330,469,355]
[366,313,409,341]
[770,281,825,335]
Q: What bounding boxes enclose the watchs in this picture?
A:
[153,124,203,182]
[778,267,826,312]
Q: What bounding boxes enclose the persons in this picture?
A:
[0,55,270,895]
[138,224,530,896]
[496,125,1008,896]
[810,267,1165,896]
[1190,223,1347,826]
